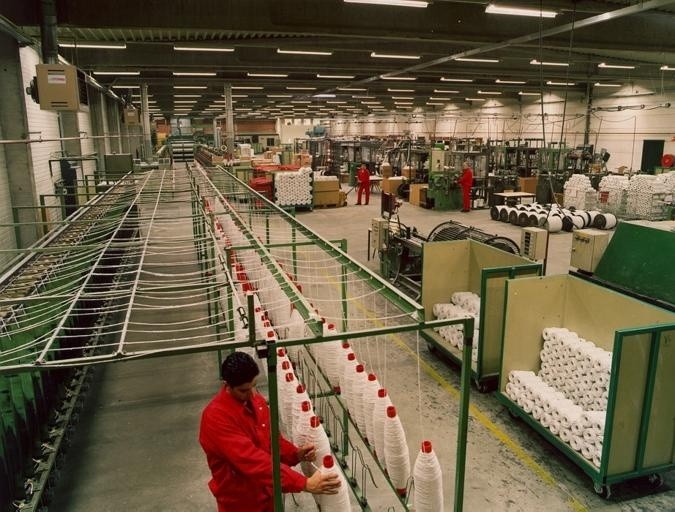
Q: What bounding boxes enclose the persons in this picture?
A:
[197,349,343,512]
[452,160,473,212]
[354,163,371,206]
